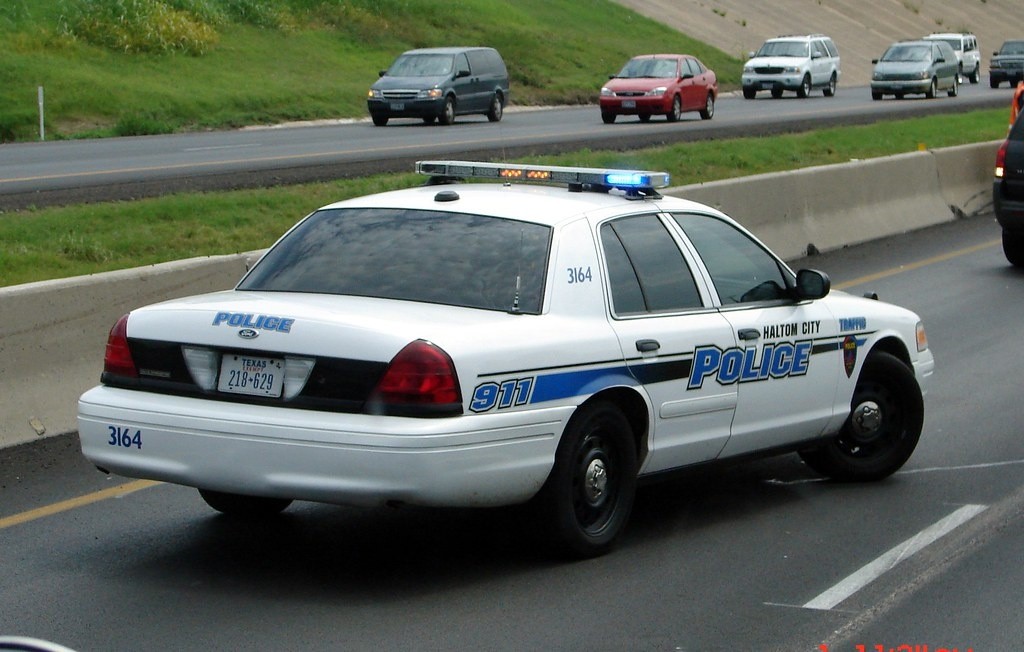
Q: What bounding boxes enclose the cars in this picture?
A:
[73,159,931,563]
[599,54,718,124]
[990,87,1024,271]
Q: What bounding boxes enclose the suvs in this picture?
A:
[743,34,843,99]
[988,40,1024,89]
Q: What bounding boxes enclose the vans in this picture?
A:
[869,40,961,100]
[368,46,510,127]
[924,31,981,84]
